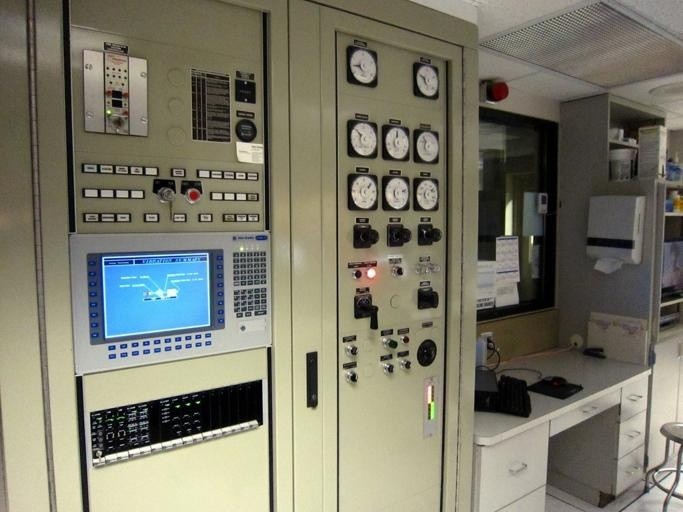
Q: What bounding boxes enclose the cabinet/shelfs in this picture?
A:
[559,96,682,343]
[475,421,547,511]
[616,375,648,496]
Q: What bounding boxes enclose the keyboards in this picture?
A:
[487,374,531,418]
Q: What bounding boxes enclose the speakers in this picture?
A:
[476,339,487,370]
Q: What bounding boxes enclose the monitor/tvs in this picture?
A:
[661,241,683,298]
[86,249,226,347]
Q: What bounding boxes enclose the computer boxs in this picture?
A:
[474,370,499,412]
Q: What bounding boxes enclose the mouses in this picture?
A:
[541,376,567,391]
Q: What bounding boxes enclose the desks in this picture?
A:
[471,347,652,448]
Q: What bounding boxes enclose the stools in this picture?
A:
[643,422,683,512]
